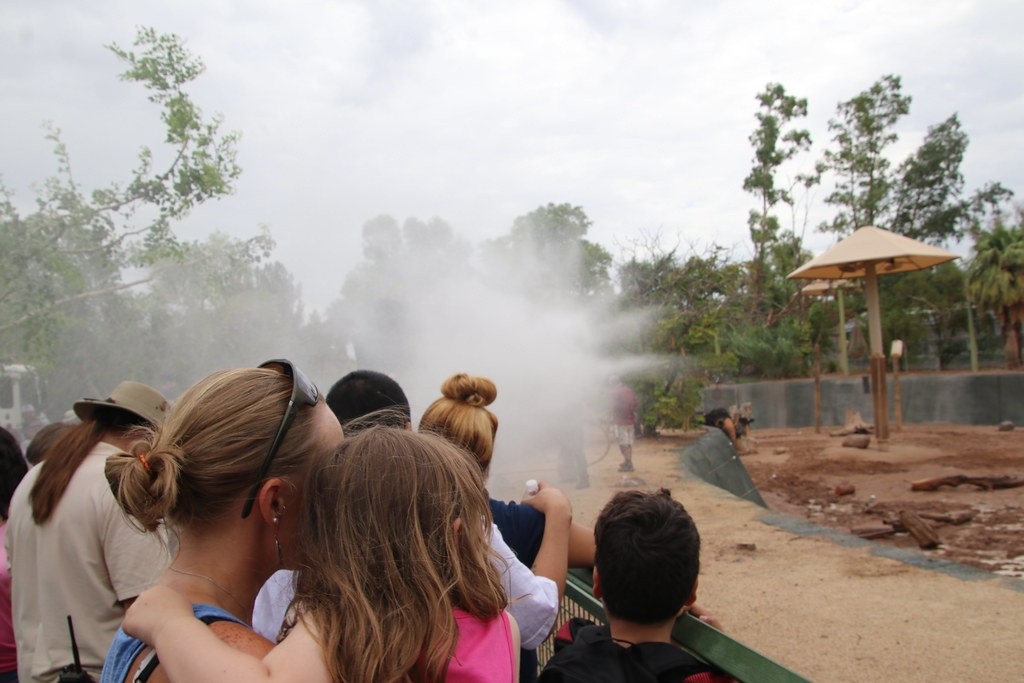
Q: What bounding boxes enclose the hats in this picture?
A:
[72,381,170,430]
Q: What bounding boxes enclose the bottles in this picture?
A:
[522,479,540,499]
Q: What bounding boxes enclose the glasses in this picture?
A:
[239,359,319,520]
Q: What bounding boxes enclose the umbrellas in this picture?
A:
[794,278,863,297]
[785,227,962,444]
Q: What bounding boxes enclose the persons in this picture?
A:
[607,373,636,471]
[0,357,727,683]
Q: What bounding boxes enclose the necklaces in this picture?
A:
[170,564,231,593]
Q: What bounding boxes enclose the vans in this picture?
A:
[0,363,103,449]
